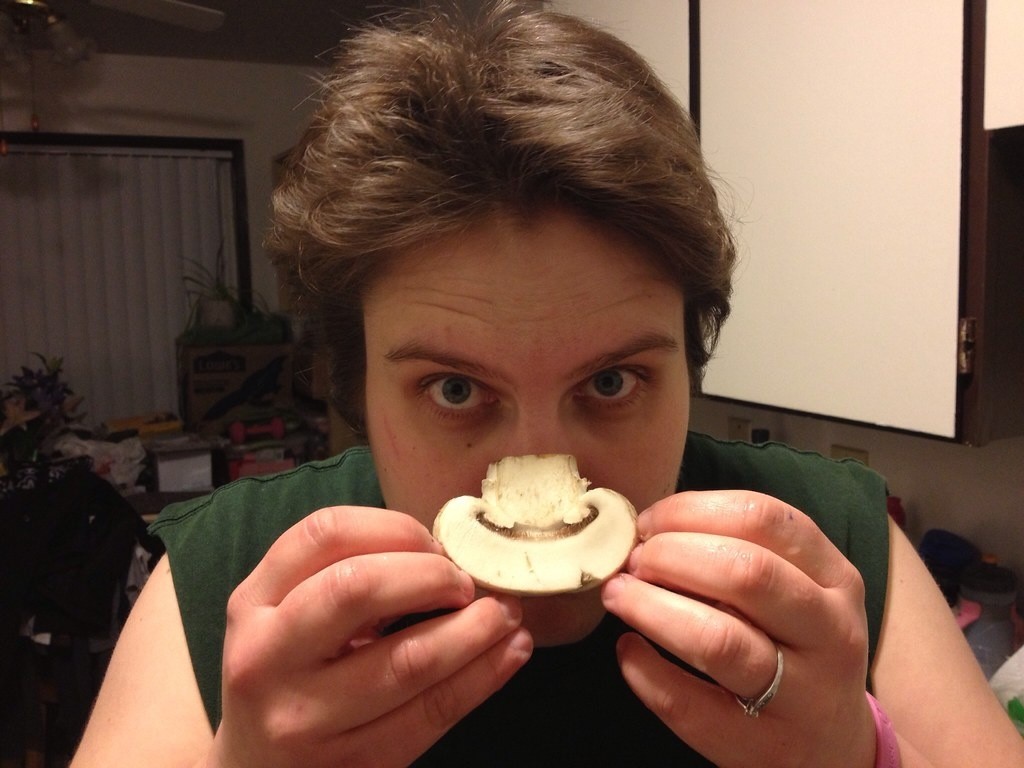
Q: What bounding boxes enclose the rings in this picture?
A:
[735,646,783,719]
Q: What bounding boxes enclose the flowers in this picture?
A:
[0,352,86,448]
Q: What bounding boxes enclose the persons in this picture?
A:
[70,0,1024,768]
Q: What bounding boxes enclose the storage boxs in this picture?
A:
[141,344,294,495]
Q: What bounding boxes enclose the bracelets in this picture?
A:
[866,692,901,768]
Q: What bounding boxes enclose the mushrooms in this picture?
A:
[433,453,638,596]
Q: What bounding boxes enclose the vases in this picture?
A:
[8,443,50,492]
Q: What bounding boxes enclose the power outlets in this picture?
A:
[728,417,752,442]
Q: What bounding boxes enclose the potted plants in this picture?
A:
[175,244,249,331]
